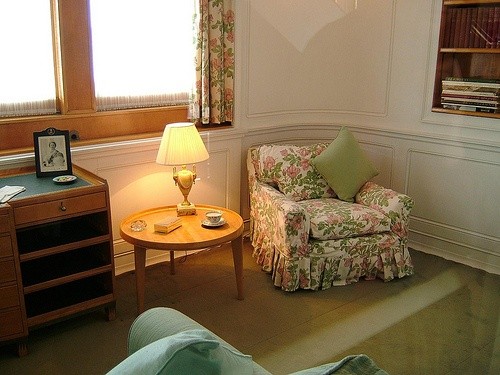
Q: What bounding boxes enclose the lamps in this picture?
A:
[156,122,210,217]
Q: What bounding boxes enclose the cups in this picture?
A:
[205,211,222,224]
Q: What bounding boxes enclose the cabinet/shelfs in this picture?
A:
[430,0,500,120]
[0,163,118,358]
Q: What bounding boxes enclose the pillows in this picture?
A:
[106,329,254,375]
[310,126,380,203]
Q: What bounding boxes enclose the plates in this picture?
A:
[53,175,77,183]
[201,218,226,227]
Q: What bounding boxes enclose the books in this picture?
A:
[440,7,478,48]
[474,7,500,48]
[440,77,500,114]
[154,217,182,233]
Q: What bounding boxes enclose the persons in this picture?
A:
[42,142,65,167]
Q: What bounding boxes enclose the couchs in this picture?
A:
[127,308,391,375]
[247,142,415,292]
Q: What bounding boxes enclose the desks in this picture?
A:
[120,204,244,315]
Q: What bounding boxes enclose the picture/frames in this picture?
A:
[33,127,72,178]
